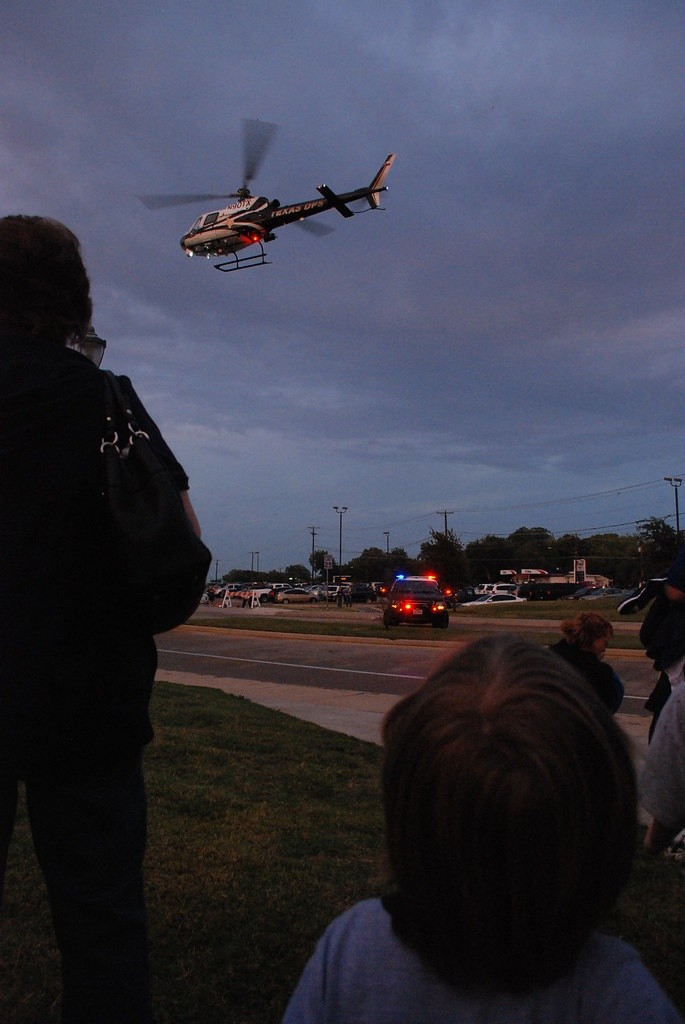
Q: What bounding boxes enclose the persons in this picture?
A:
[282,577,683,1024]
[1,213,212,1023]
[335,586,353,608]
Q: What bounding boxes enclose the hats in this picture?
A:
[0,217,93,338]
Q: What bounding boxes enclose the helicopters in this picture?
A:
[137,119,397,273]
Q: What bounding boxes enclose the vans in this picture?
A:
[464,583,578,602]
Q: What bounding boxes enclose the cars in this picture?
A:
[461,594,526,611]
[206,581,387,605]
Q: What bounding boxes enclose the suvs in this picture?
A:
[379,575,453,628]
